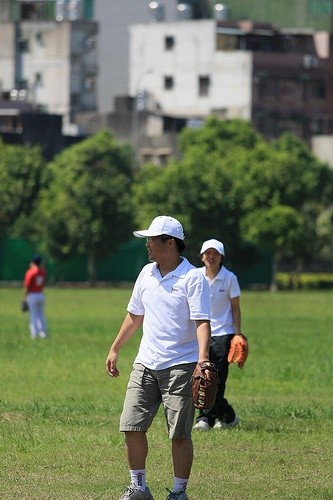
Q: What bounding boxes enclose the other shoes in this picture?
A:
[212,415,240,429]
[120,486,154,500]
[163,488,188,500]
[194,420,209,431]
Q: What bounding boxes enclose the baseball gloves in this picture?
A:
[227,333,249,369]
[22,302,29,312]
[191,361,220,410]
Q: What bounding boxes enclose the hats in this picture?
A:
[133,215,185,240]
[200,239,225,256]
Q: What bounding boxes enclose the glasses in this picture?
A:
[146,237,161,243]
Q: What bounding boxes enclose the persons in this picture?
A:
[23,255,48,340]
[193,239,248,431]
[106,216,220,500]
